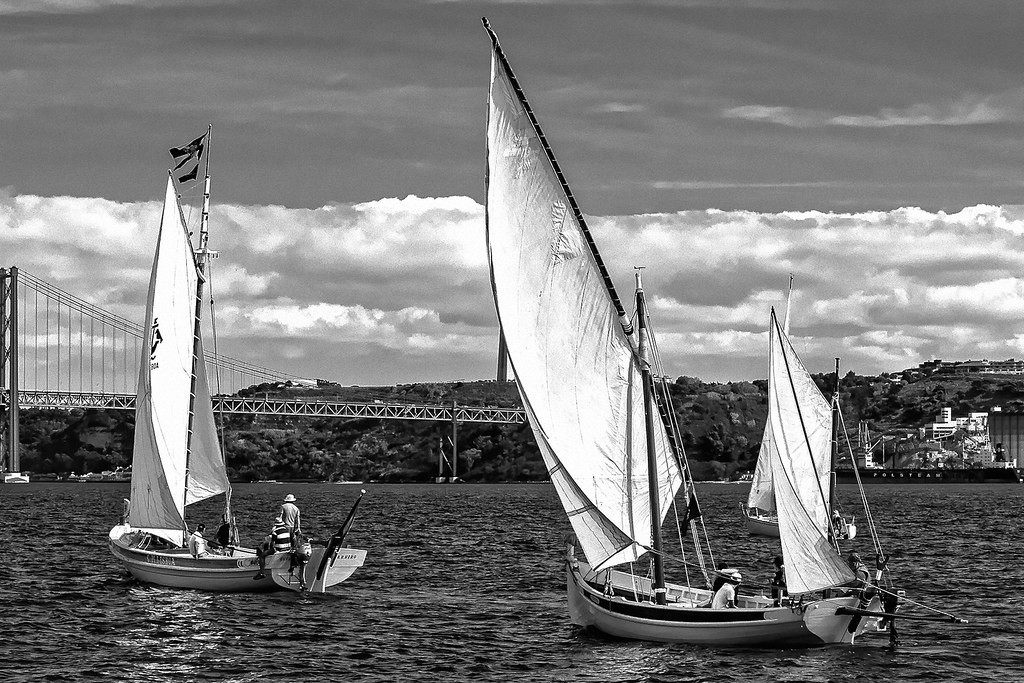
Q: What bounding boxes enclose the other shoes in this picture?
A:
[253,572,266,580]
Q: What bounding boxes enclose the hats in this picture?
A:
[848,553,860,561]
[775,556,783,569]
[198,524,205,531]
[274,517,285,525]
[731,573,742,581]
[833,510,839,514]
[284,494,296,501]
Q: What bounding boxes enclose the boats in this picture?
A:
[828,403,1017,482]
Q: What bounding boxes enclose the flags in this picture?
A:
[170,133,208,182]
[677,493,701,539]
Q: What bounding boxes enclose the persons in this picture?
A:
[711,562,742,611]
[252,493,301,580]
[835,552,871,598]
[771,557,790,607]
[188,524,211,560]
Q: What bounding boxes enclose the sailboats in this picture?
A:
[106,121,368,596]
[741,273,855,552]
[478,13,970,648]
